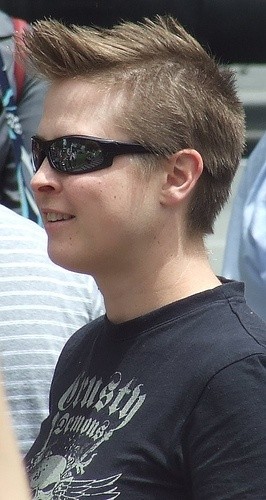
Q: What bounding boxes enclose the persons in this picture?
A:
[0,10,265,500]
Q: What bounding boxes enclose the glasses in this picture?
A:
[29,135,178,173]
